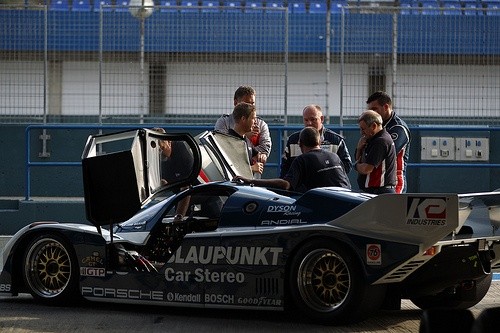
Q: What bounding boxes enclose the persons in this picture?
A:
[232,126,352,193]
[355,90,412,194]
[215,85,272,179]
[150,127,223,225]
[280,103,353,179]
[354,110,398,194]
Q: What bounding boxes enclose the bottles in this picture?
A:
[254,159,261,179]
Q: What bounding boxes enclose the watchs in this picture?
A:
[175,213,183,220]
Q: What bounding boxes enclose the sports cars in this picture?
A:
[0,127,500,326]
